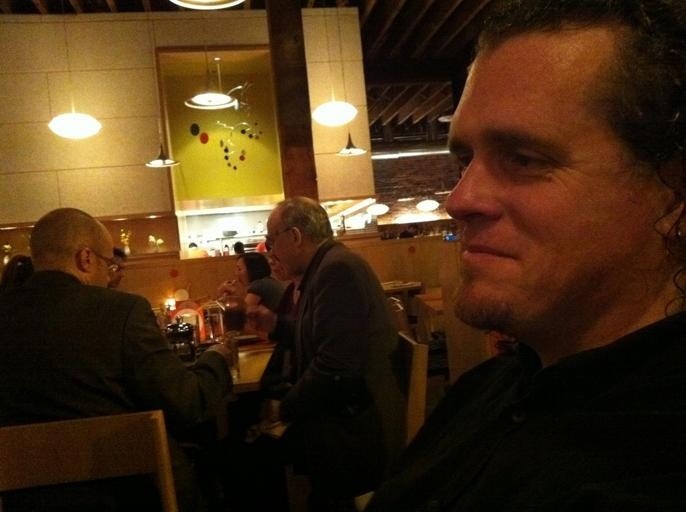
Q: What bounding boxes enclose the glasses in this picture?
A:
[91,248,126,276]
[266,225,291,247]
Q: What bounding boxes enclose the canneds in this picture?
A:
[203,303,225,344]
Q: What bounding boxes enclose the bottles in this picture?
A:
[165,316,196,364]
[203,307,226,344]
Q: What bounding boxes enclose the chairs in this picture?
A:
[2,410,182,512]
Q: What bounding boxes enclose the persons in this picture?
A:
[219,251,283,310]
[238,197,406,511]
[1,207,234,512]
[188,241,265,256]
[211,196,404,510]
[367,0,686,511]
[2,253,32,283]
[108,246,129,290]
[240,236,301,343]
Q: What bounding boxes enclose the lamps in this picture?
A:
[184,41,237,113]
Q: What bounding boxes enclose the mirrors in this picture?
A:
[153,41,283,213]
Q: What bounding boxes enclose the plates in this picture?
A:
[235,334,259,344]
